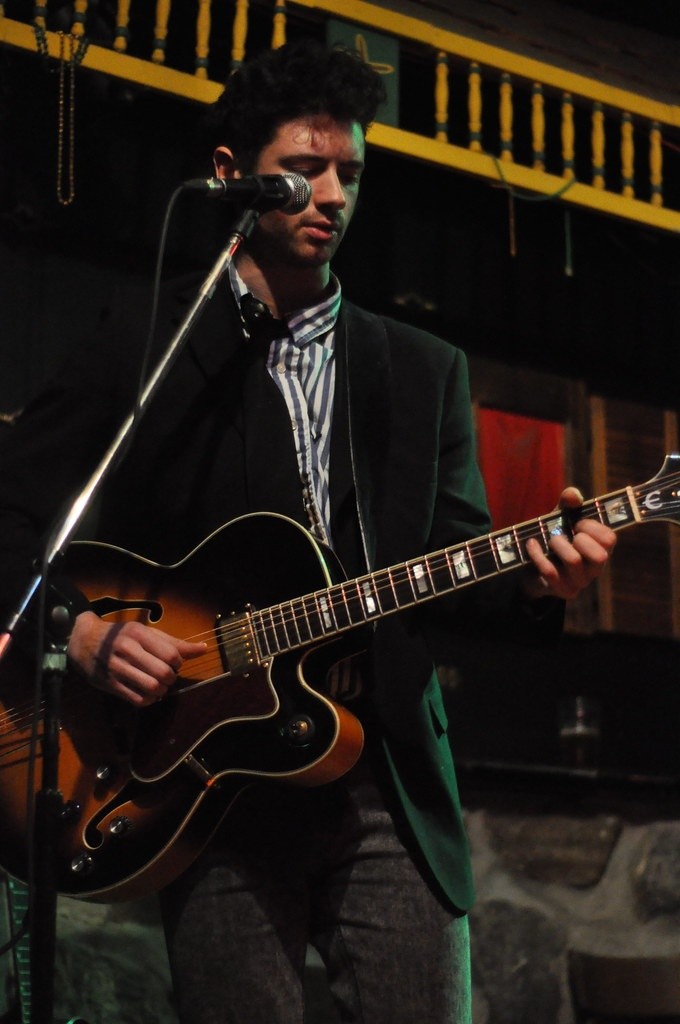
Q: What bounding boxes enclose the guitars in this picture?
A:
[1,448,680,907]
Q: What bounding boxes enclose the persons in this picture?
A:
[0,40,617,1024]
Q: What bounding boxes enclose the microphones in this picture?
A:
[186,170,312,213]
